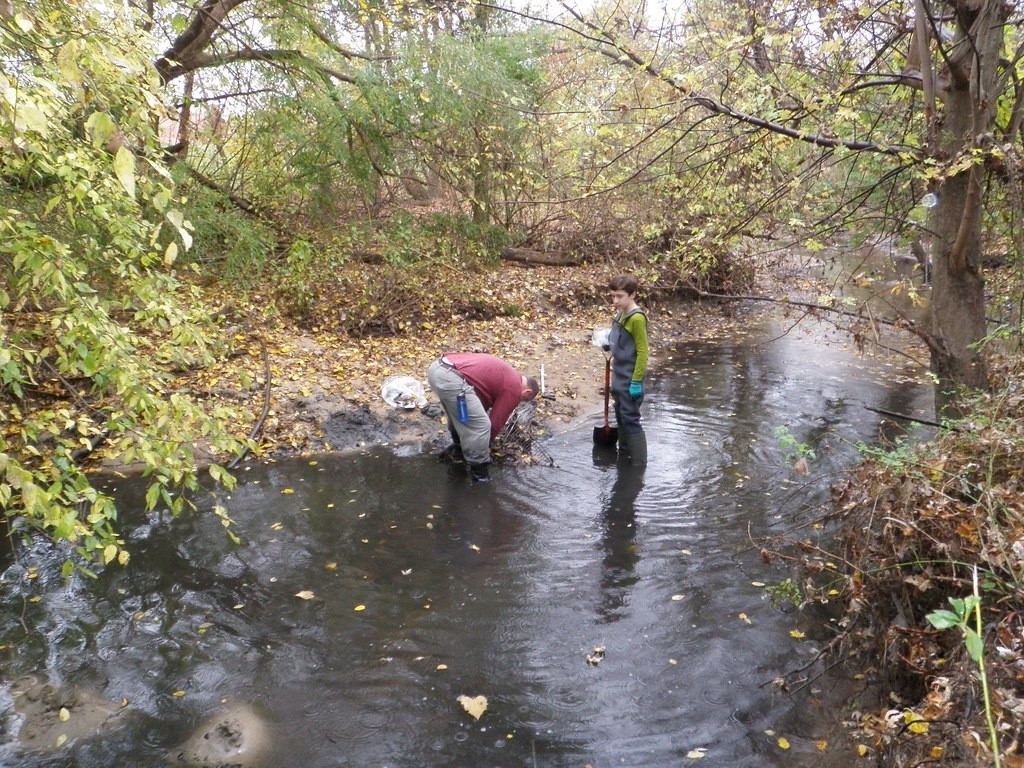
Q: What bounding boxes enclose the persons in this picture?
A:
[427,353,539,481]
[603,275,648,435]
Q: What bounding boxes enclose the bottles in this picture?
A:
[457,393,468,422]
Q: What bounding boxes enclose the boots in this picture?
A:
[618,426,626,447]
[471,463,490,481]
[624,431,647,464]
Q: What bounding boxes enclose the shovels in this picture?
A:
[594,343,619,446]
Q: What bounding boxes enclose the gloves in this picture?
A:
[629,380,642,400]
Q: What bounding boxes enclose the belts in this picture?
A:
[439,358,464,379]
